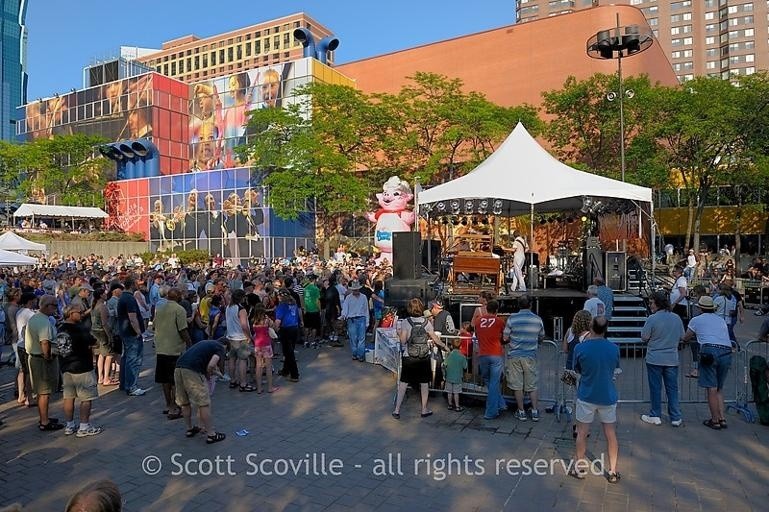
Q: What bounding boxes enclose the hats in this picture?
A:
[80,283,95,292]
[427,300,444,309]
[696,295,719,310]
[348,281,363,290]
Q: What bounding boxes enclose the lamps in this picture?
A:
[539,213,585,225]
[442,215,490,226]
[422,199,504,215]
[581,197,635,215]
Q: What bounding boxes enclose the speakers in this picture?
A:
[605,251,626,291]
[584,249,602,293]
[422,239,441,273]
[392,231,422,279]
[459,302,483,330]
[390,281,428,316]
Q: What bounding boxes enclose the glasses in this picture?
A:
[45,303,58,308]
[70,311,82,314]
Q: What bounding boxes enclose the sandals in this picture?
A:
[703,417,728,430]
[38,418,65,432]
[207,432,226,444]
[447,404,466,412]
[186,425,202,438]
[229,379,280,395]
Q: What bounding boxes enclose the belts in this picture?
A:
[29,353,43,357]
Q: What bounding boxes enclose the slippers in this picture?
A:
[163,410,184,420]
[685,373,699,379]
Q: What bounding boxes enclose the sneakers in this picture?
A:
[129,389,146,397]
[514,408,528,422]
[568,470,586,480]
[329,341,344,347]
[641,414,663,426]
[64,425,102,438]
[606,470,618,484]
[671,418,683,427]
[420,410,433,417]
[527,408,539,422]
[392,411,400,419]
[277,369,300,382]
[303,342,322,348]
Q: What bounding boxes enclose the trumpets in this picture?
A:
[217,201,250,216]
[166,204,182,231]
[150,211,158,222]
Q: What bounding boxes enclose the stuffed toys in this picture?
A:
[365,175,416,267]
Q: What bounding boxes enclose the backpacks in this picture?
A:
[406,316,432,359]
